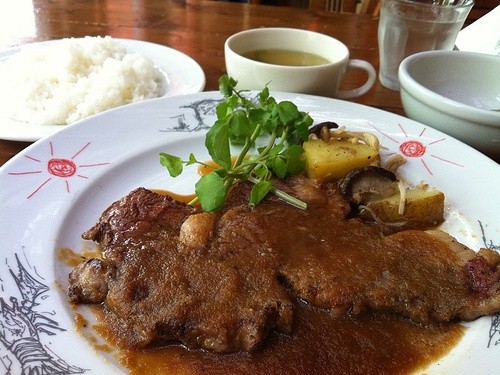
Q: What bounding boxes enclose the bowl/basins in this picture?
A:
[397,51,500,153]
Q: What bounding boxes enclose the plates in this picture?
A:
[0,40,204,141]
[0,90,500,375]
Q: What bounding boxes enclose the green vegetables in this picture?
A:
[159,75,313,211]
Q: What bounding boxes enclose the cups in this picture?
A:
[378,0,474,90]
[224,28,376,98]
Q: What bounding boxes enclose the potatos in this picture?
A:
[366,188,444,223]
[301,139,380,184]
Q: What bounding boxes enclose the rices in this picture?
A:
[0,35,167,127]
